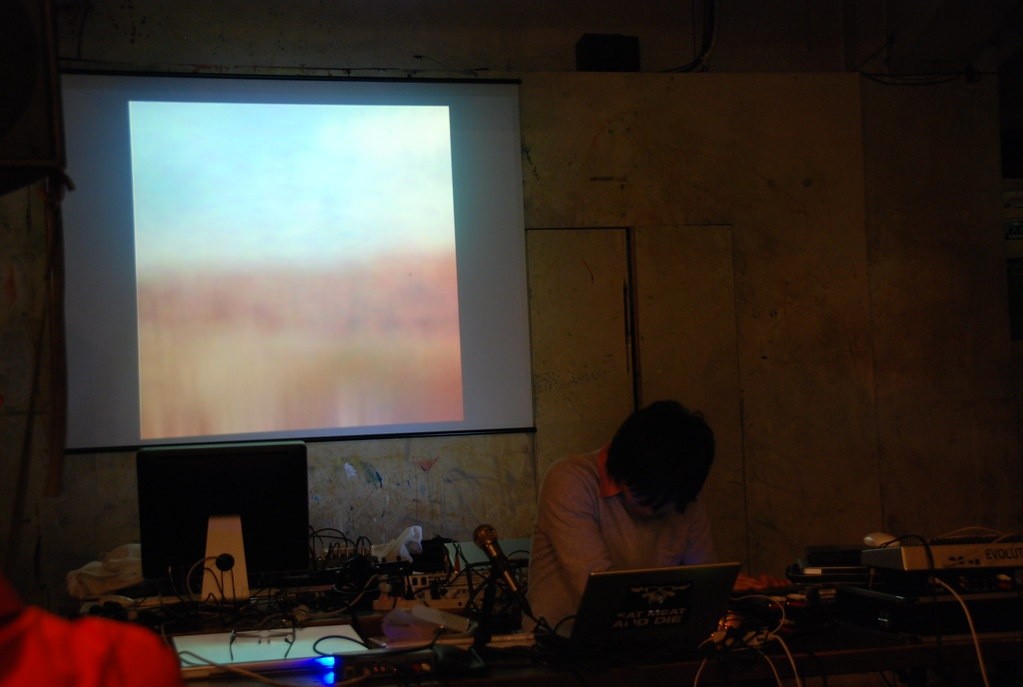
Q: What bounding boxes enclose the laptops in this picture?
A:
[543,563,740,669]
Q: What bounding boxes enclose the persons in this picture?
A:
[0,577,187,687]
[522,399,720,634]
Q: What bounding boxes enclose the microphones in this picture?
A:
[474,523,533,619]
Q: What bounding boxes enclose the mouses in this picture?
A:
[864,532,900,548]
[98,595,132,609]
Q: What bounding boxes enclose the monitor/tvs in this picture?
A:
[139,439,312,603]
